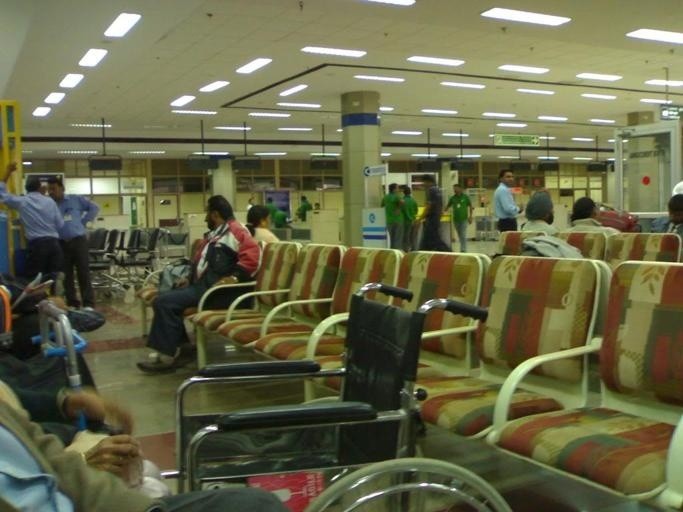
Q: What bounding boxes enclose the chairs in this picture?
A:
[88,225,171,309]
[134,229,681,508]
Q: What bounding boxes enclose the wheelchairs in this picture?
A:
[161,281,512,511]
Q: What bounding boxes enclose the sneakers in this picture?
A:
[173,344,198,367]
[65,306,105,332]
[135,355,176,374]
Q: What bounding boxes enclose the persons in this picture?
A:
[382,166,474,254]
[659,193,683,245]
[493,169,523,233]
[519,190,560,235]
[564,197,622,236]
[648,182,683,233]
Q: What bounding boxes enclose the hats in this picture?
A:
[525,193,553,221]
[671,181,683,197]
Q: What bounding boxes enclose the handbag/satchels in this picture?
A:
[156,259,195,295]
[204,242,239,287]
